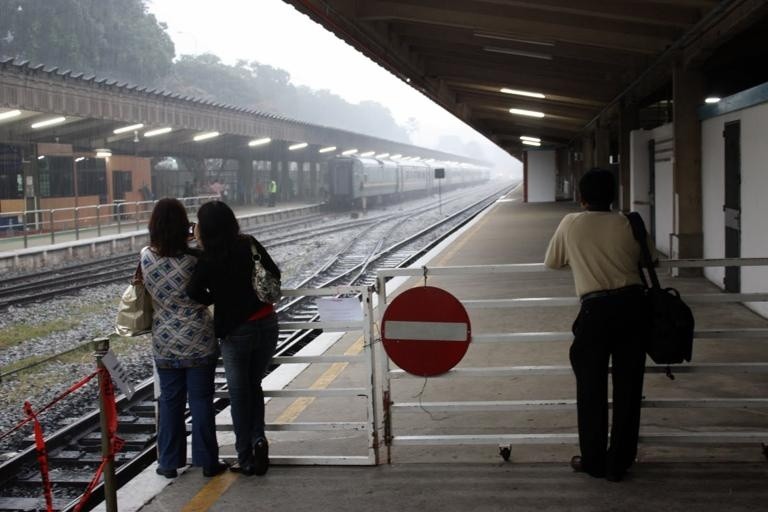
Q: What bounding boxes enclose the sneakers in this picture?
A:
[229,461,255,475]
[570,455,633,483]
[155,466,180,478]
[201,458,228,478]
[252,438,269,477]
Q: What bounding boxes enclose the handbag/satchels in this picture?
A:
[250,260,283,307]
[114,262,154,338]
[644,282,695,366]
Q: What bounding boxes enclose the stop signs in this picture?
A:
[380,284,471,378]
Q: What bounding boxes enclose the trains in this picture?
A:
[324,153,491,211]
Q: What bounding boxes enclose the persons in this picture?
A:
[541,166,659,480]
[186,199,283,477]
[182,174,281,213]
[137,197,233,480]
